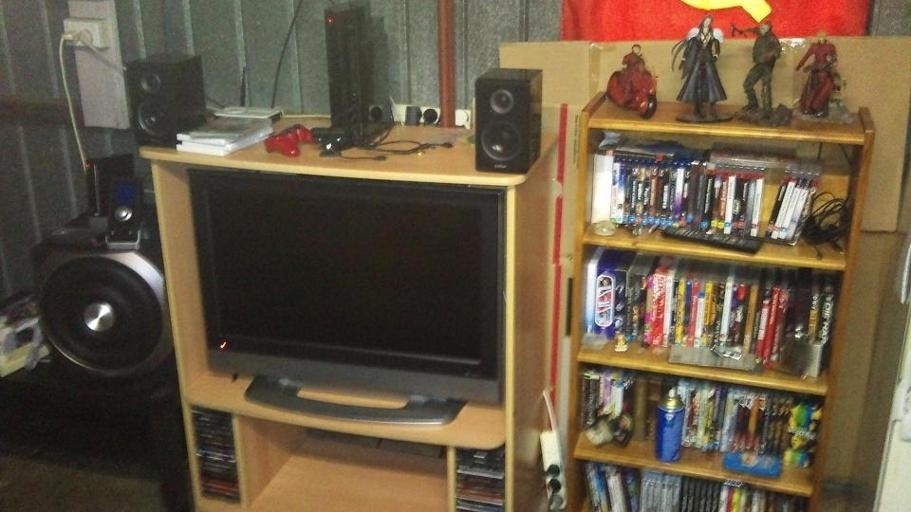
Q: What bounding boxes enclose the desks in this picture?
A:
[2,356,194,511]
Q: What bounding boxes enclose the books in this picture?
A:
[581,364,824,469]
[584,462,805,512]
[176,116,274,156]
[591,138,823,245]
[585,247,838,378]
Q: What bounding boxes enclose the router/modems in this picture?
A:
[83,155,134,215]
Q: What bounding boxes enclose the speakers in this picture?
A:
[474,67,543,175]
[30,203,181,391]
[125,51,206,148]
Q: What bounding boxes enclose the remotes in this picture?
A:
[662,226,762,254]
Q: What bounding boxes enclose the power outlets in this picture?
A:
[62,16,108,49]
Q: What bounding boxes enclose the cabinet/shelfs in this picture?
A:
[562,88,876,512]
[139,118,559,512]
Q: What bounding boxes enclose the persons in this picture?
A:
[670,16,728,118]
[732,20,782,111]
[798,28,839,118]
[604,44,658,119]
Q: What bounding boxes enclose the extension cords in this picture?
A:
[539,431,568,510]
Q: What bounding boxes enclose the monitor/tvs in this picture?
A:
[185,168,503,427]
